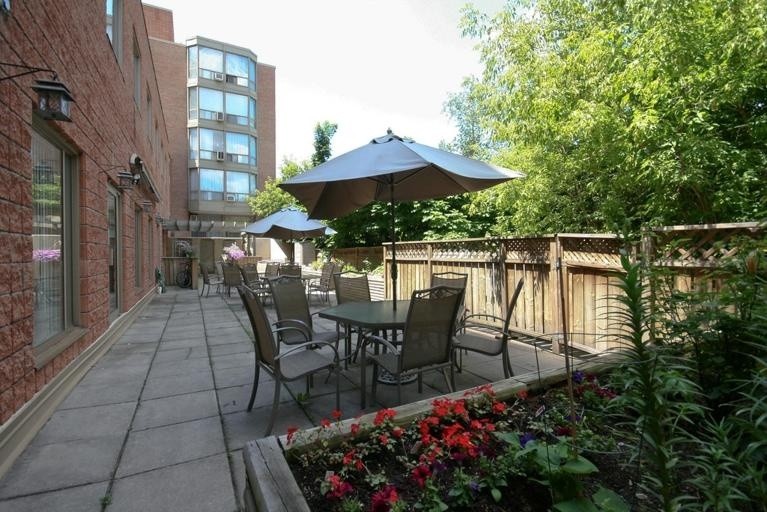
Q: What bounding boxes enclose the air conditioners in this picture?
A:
[217,111,225,122]
[227,193,235,202]
[215,72,224,82]
[217,150,226,161]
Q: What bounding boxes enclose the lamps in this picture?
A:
[1,62,75,122]
[101,163,134,192]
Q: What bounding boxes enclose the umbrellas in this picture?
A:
[238,202,339,264]
[273,121,528,349]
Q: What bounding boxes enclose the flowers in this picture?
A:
[224,243,244,260]
